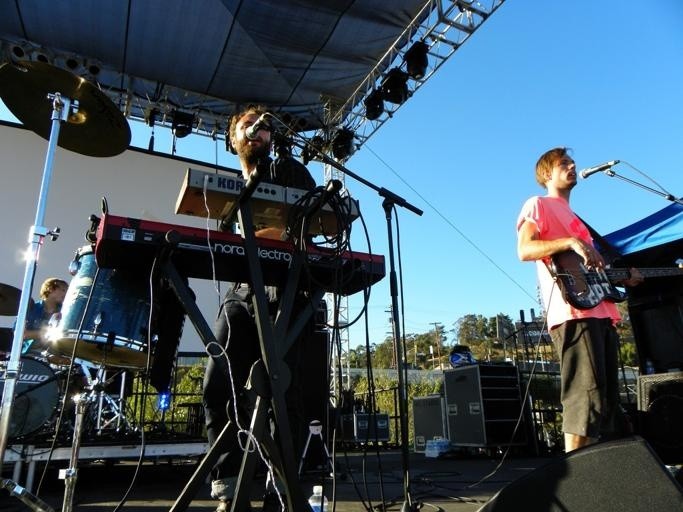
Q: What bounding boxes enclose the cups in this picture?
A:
[424,441,439,458]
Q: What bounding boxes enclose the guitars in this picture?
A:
[550,250,683,309]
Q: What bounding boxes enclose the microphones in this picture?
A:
[245,112,270,141]
[579,159,621,180]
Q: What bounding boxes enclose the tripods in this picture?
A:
[79,362,138,435]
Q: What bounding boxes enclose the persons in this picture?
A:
[517,148,644,454]
[449,344,472,368]
[204,107,330,512]
[13,279,69,364]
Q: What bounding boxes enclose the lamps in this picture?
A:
[6,34,109,83]
[168,106,324,167]
[329,38,431,164]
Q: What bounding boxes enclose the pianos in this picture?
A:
[95,215,385,296]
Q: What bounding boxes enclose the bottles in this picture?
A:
[642,361,654,374]
[427,438,451,453]
[308,485,327,512]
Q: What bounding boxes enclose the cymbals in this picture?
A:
[0,61,130,157]
[0,284,34,316]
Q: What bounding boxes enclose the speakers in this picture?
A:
[636,372,683,464]
[478,434,682,511]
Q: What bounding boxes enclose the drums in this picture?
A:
[0,354,61,438]
[72,359,134,398]
[45,245,159,370]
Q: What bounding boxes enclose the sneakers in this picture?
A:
[262,493,315,512]
[213,499,250,512]
